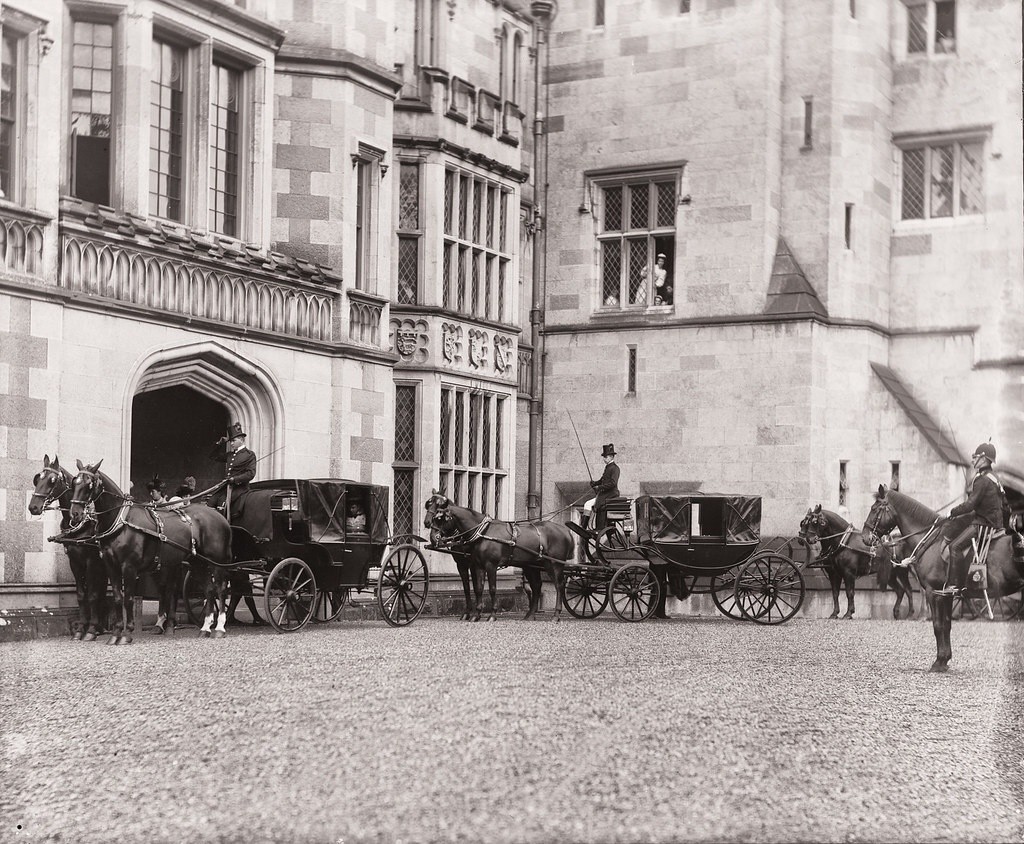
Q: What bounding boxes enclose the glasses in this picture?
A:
[972,454,979,459]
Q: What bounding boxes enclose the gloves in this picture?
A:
[218,437,228,446]
[593,485,599,491]
[590,481,595,487]
[951,508,958,517]
[227,476,235,485]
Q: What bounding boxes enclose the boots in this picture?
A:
[932,548,965,597]
[581,515,590,530]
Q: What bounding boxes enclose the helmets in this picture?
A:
[973,436,996,464]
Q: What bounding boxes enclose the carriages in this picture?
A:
[422,488,809,626]
[27,454,432,634]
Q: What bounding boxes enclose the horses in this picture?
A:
[797,484,1023,674]
[28,454,234,645]
[424,487,575,623]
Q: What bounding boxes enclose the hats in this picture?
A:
[176,477,196,497]
[601,443,617,456]
[226,423,247,441]
[146,473,167,493]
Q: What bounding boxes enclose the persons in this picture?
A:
[579,443,620,535]
[344,500,367,531]
[147,478,168,508]
[604,253,674,306]
[207,423,256,521]
[167,477,196,510]
[933,437,1004,598]
